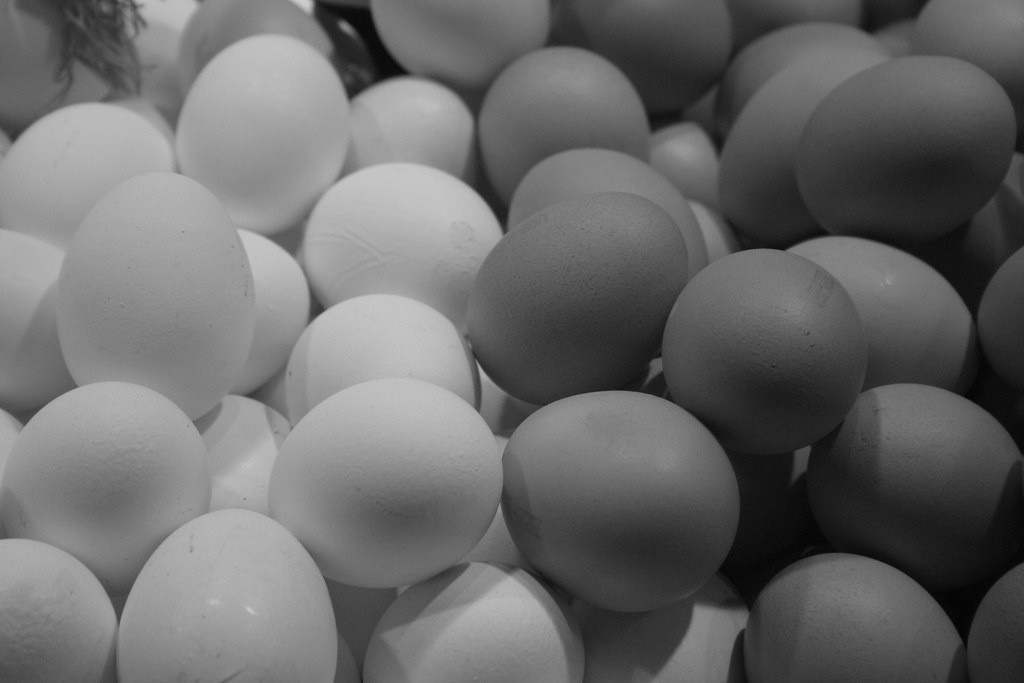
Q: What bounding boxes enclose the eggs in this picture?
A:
[0,0,1024,683]
